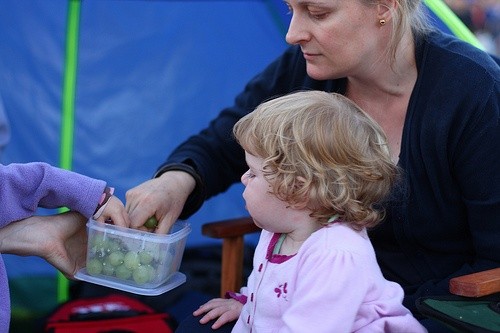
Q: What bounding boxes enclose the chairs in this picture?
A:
[200,211,500,333]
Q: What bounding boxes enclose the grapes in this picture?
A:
[87,216,158,284]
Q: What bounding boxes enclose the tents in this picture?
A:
[0,0,489,333]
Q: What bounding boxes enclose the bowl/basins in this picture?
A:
[86,216,191,288]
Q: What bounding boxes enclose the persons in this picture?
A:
[0,158,129,333]
[1,208,94,281]
[193,88,431,333]
[119,0,499,333]
[440,0,500,63]
